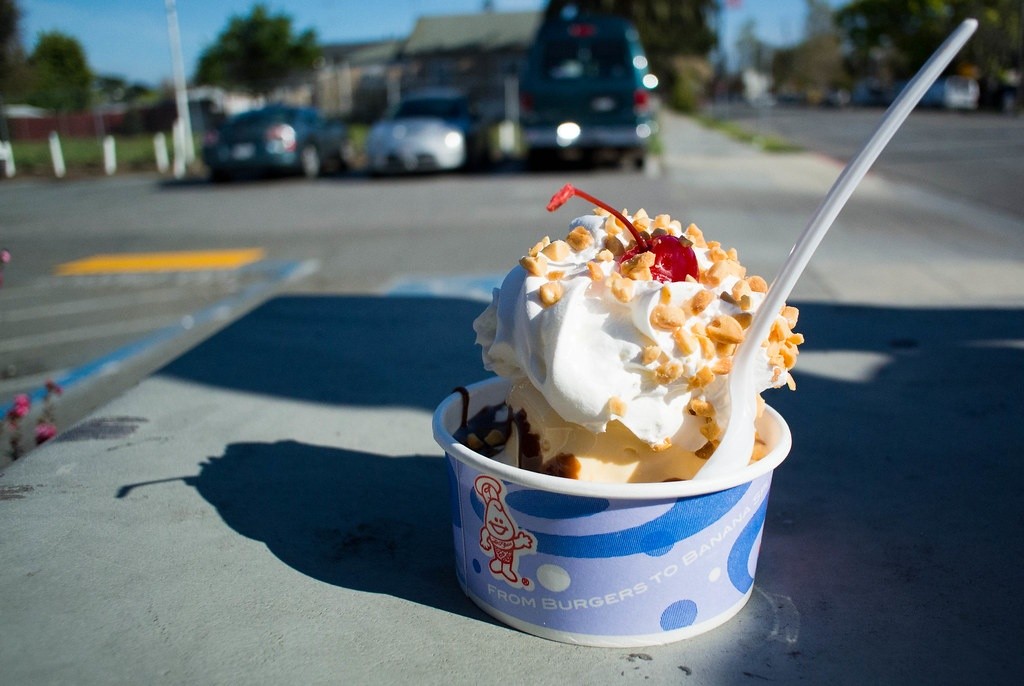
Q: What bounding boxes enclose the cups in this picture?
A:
[434,375,792,648]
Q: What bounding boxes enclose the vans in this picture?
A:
[513,11,670,172]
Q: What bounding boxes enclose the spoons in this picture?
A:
[687,17,978,479]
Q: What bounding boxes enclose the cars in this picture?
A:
[364,83,489,179]
[772,68,1024,117]
[200,102,350,182]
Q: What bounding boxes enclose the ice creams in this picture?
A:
[449,183,805,491]
